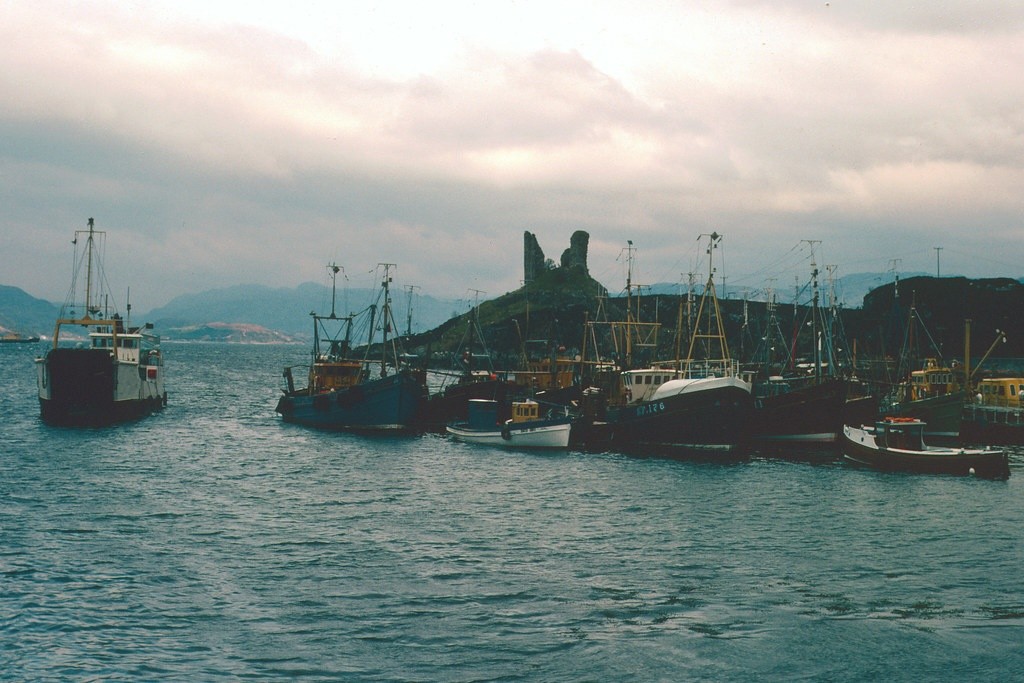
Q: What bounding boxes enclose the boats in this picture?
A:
[35,217,168,429]
[423,230,1024,448]
[446,400,572,450]
[274,262,422,434]
[842,417,1011,479]
